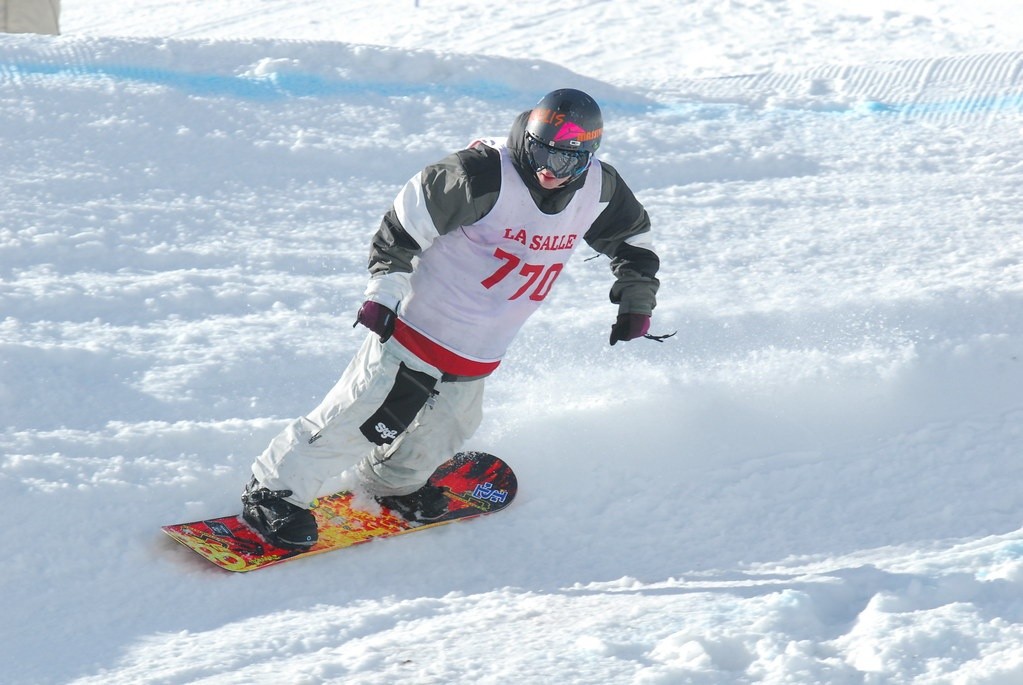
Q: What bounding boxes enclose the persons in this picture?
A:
[242,88,661,547]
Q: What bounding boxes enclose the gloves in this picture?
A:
[357,300,396,344]
[609,314,651,345]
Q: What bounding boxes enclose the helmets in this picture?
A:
[524,89,602,153]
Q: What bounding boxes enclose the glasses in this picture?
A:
[525,134,589,179]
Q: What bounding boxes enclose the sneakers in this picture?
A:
[241,471,318,548]
[374,478,448,523]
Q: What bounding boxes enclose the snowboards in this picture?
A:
[161,450,517,573]
